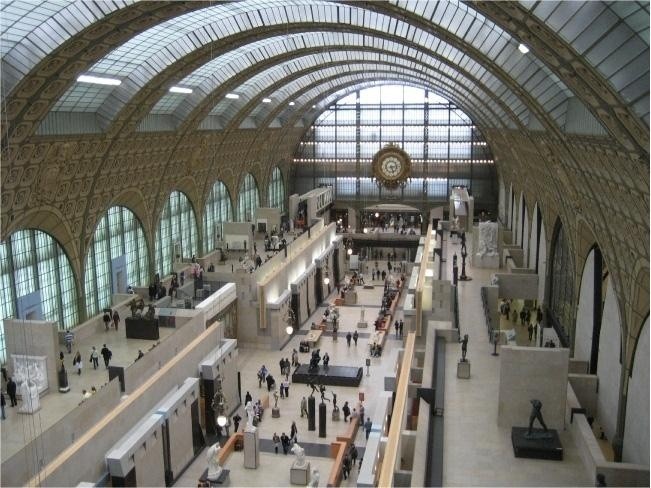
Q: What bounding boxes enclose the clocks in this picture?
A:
[371,144,412,189]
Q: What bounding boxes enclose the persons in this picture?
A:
[0,209,423,487]
[499,297,543,340]
[527,399,548,435]
[458,334,469,362]
